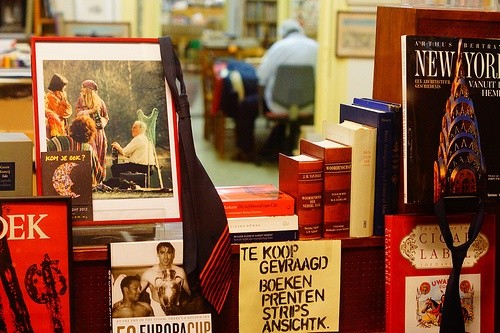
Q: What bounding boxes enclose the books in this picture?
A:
[384,213,497,333]
[400,35,500,212]
[278,95,403,240]
[214,185,299,242]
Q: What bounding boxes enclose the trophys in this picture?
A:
[155,269,186,308]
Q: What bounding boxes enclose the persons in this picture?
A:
[75,79,111,185]
[48,117,106,194]
[45,73,74,140]
[113,276,155,318]
[261,18,321,161]
[111,121,156,177]
[112,242,191,317]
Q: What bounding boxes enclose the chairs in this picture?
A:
[201,51,242,159]
[263,64,317,163]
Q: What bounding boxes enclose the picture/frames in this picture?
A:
[61,21,132,38]
[0,196,74,333]
[337,9,377,60]
[30,33,183,227]
[73,0,118,23]
[0,33,32,77]
